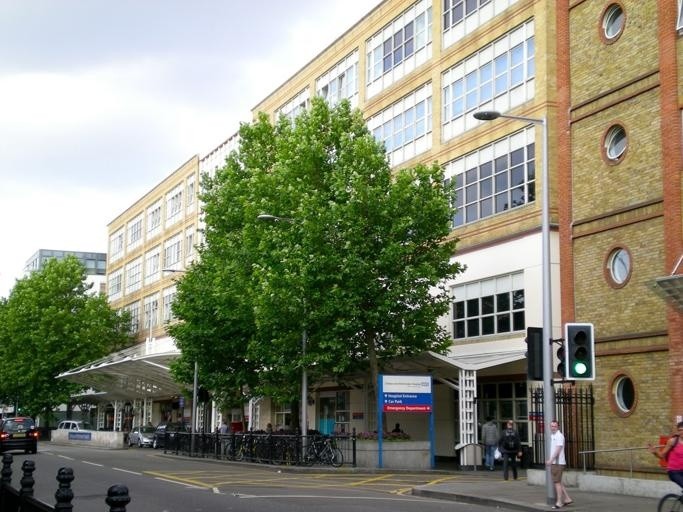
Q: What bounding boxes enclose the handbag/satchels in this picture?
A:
[494,448,502,462]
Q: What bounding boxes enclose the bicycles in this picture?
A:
[657,490,683,512]
[225,429,343,468]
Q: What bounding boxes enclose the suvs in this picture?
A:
[153,422,199,452]
[0,417,39,453]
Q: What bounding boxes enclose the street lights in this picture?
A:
[475,111,557,503]
[258,215,308,464]
[163,269,200,456]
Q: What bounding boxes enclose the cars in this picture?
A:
[127,426,155,448]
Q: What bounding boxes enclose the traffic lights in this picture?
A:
[557,323,596,380]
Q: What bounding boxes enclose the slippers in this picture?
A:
[552,501,573,509]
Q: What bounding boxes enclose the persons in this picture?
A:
[480,415,498,470]
[496,419,523,480]
[544,419,573,509]
[216,421,228,433]
[166,409,172,422]
[177,412,183,423]
[391,423,402,433]
[644,420,682,491]
[265,423,272,434]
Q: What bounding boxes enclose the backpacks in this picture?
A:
[503,429,519,451]
[665,435,680,461]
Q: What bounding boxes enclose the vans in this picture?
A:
[58,420,91,430]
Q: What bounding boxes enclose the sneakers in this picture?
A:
[487,464,495,471]
